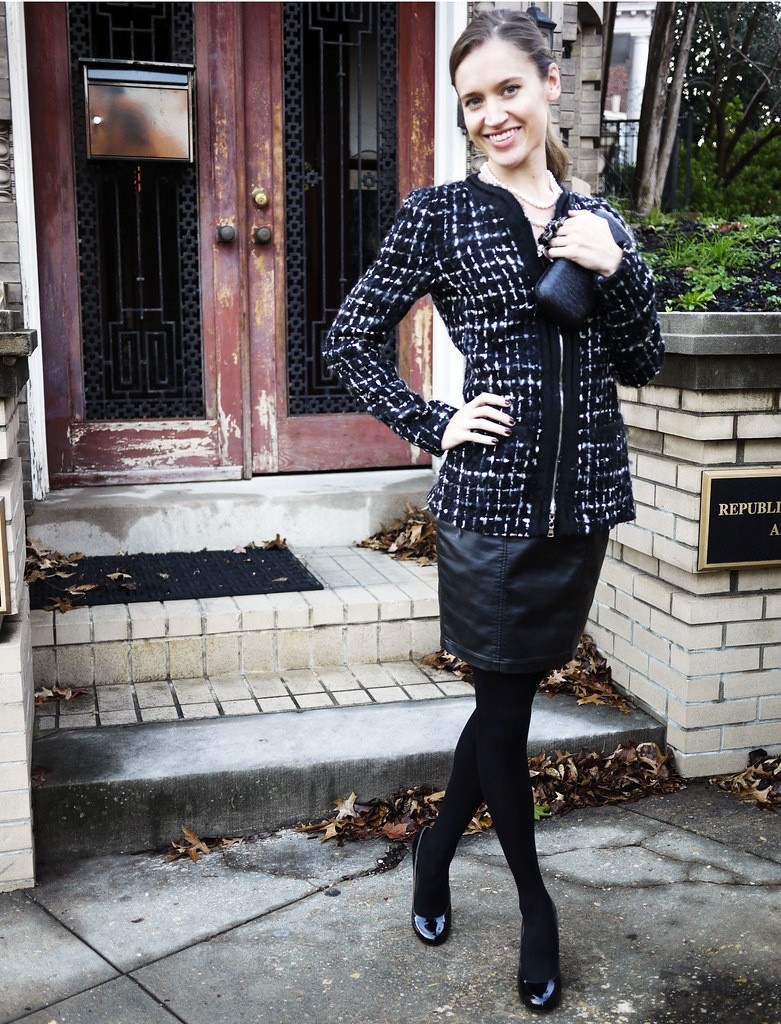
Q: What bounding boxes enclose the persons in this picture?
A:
[324,9,666,1009]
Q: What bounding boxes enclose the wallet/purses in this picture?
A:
[534,208,632,325]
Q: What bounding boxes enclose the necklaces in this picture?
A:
[481,160,562,229]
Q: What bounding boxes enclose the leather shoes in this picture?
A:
[518,901,562,1013]
[411,826,452,946]
